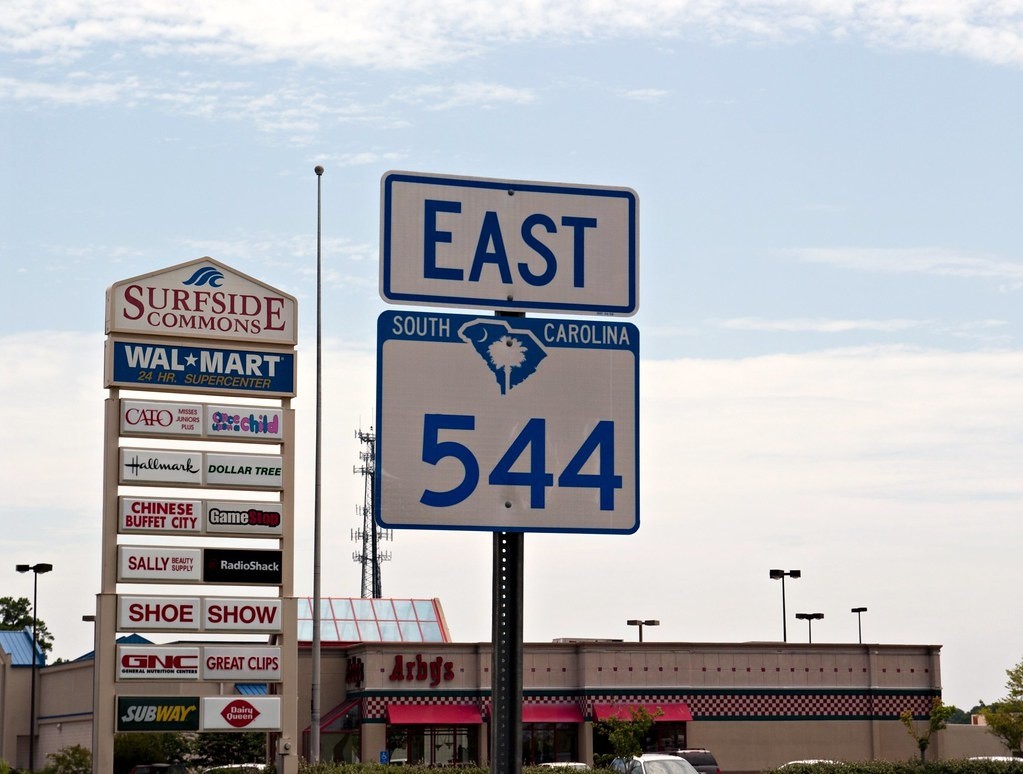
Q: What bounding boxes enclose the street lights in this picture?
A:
[627,620,660,642]
[852,607,867,644]
[770,570,801,643]
[16,563,53,774]
[795,613,824,643]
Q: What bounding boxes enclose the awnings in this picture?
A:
[487,703,585,722]
[592,703,693,722]
[386,704,483,724]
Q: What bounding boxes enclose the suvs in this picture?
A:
[660,747,720,774]
[608,754,700,774]
